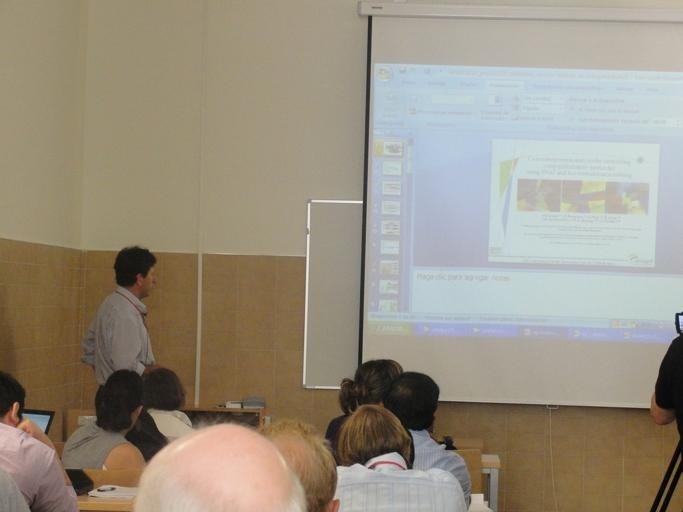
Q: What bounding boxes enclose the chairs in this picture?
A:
[449,445,483,496]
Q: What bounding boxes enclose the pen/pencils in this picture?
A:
[98,487,116,491]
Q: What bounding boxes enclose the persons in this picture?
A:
[63,370,146,471]
[328,403,468,511]
[325,358,403,448]
[0,369,78,511]
[258,415,340,511]
[131,415,308,512]
[80,245,159,386]
[126,369,193,464]
[650,332,683,465]
[384,371,471,502]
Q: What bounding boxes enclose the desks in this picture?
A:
[477,452,502,512]
[65,468,143,512]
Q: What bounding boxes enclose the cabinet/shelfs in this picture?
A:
[182,406,265,435]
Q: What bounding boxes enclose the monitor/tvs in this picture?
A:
[21,409,55,435]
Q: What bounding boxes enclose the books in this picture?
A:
[89,485,135,499]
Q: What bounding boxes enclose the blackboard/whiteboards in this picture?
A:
[302,199,364,390]
